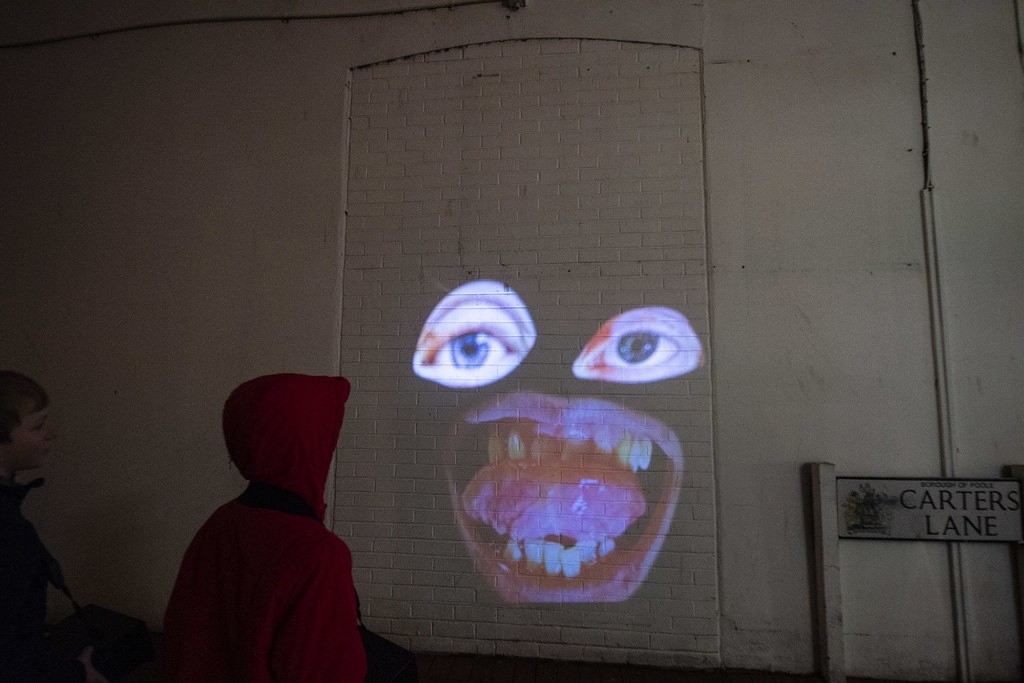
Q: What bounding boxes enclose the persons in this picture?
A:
[161,372,368,683]
[0,369,111,683]
